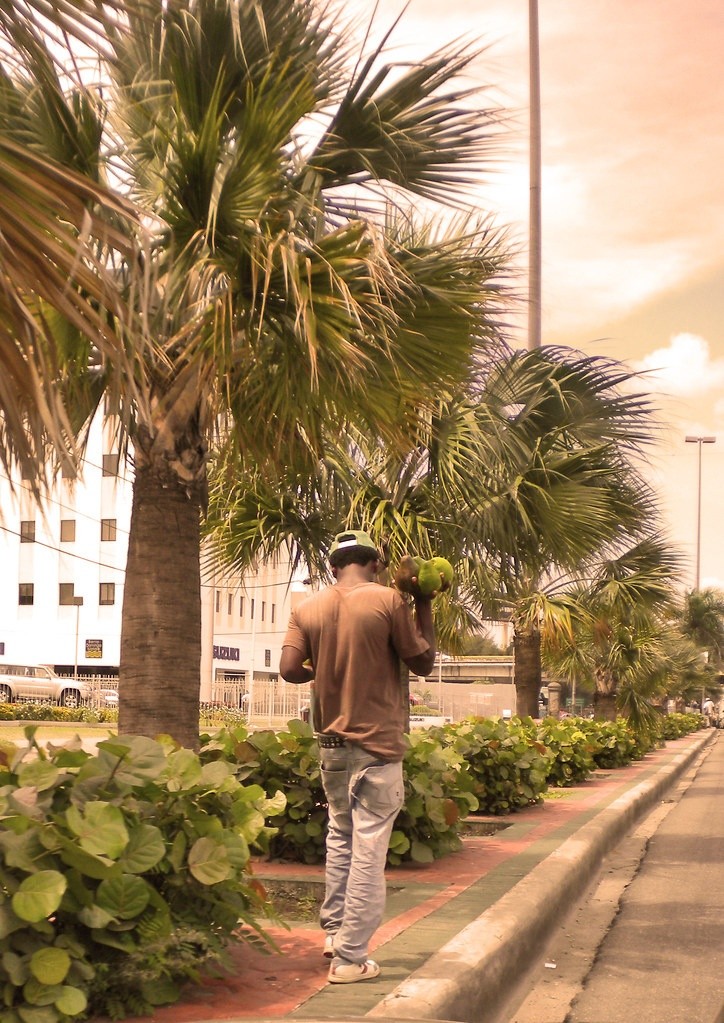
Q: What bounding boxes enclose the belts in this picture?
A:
[317,735,345,749]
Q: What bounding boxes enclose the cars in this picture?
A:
[0,666,119,709]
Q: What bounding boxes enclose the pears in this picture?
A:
[394,556,454,596]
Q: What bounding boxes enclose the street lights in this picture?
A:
[686,436,716,594]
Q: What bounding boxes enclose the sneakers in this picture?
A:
[321,935,335,959]
[327,955,382,984]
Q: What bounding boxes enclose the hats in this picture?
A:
[328,530,378,557]
[705,697,711,700]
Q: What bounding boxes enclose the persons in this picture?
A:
[280,529,452,984]
[703,698,715,716]
